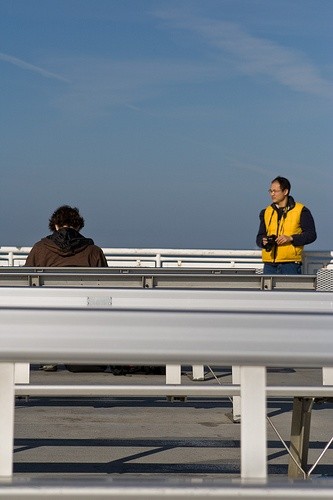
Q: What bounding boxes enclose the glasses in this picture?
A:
[268,189,283,193]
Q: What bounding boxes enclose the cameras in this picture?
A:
[263,235,277,252]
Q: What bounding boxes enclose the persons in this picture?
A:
[256,177,317,275]
[25,205,108,267]
